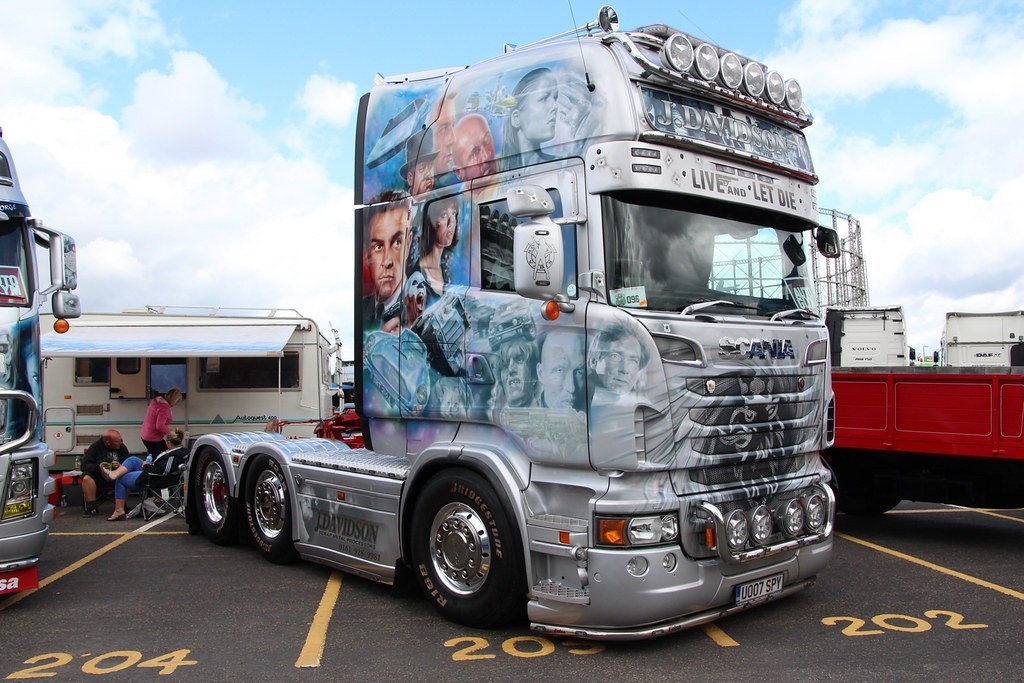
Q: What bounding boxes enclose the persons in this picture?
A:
[102,428,189,521]
[141,389,183,462]
[23,340,40,405]
[496,327,585,412]
[582,319,651,393]
[362,68,558,334]
[81,429,131,518]
[265,421,282,435]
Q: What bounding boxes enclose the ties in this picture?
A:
[375,303,385,330]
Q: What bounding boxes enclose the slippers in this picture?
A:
[101,466,115,483]
[106,513,126,521]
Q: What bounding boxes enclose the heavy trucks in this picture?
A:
[174,6,846,643]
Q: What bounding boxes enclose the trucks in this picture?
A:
[825,361,1024,523]
[0,136,83,609]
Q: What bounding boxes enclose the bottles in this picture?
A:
[75,455,81,471]
[146,454,152,462]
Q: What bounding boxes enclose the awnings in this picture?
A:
[41,323,297,433]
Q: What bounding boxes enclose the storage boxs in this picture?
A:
[49,473,61,507]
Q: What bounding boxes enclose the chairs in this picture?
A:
[81,449,128,514]
[131,471,186,521]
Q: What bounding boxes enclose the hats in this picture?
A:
[399,127,440,180]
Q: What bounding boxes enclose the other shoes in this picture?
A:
[82,506,99,519]
